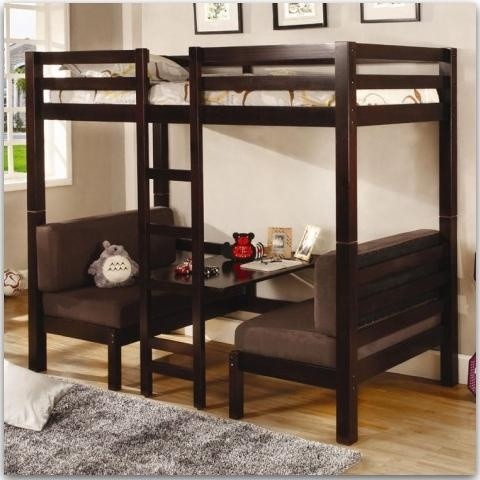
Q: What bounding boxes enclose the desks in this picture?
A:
[140,245,324,405]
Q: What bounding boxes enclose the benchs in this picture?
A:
[227,226,448,448]
[35,202,258,400]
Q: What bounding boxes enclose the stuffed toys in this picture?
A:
[88,240,139,290]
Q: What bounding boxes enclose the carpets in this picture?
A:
[3,358,366,477]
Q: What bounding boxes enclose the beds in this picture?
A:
[22,41,459,131]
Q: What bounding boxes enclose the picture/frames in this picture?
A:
[266,226,293,260]
[271,3,329,30]
[359,2,421,25]
[192,3,244,35]
[295,226,321,261]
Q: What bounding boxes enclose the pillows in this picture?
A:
[2,360,77,436]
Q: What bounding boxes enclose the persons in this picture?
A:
[276,236,284,247]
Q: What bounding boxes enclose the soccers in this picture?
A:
[4,267,24,296]
